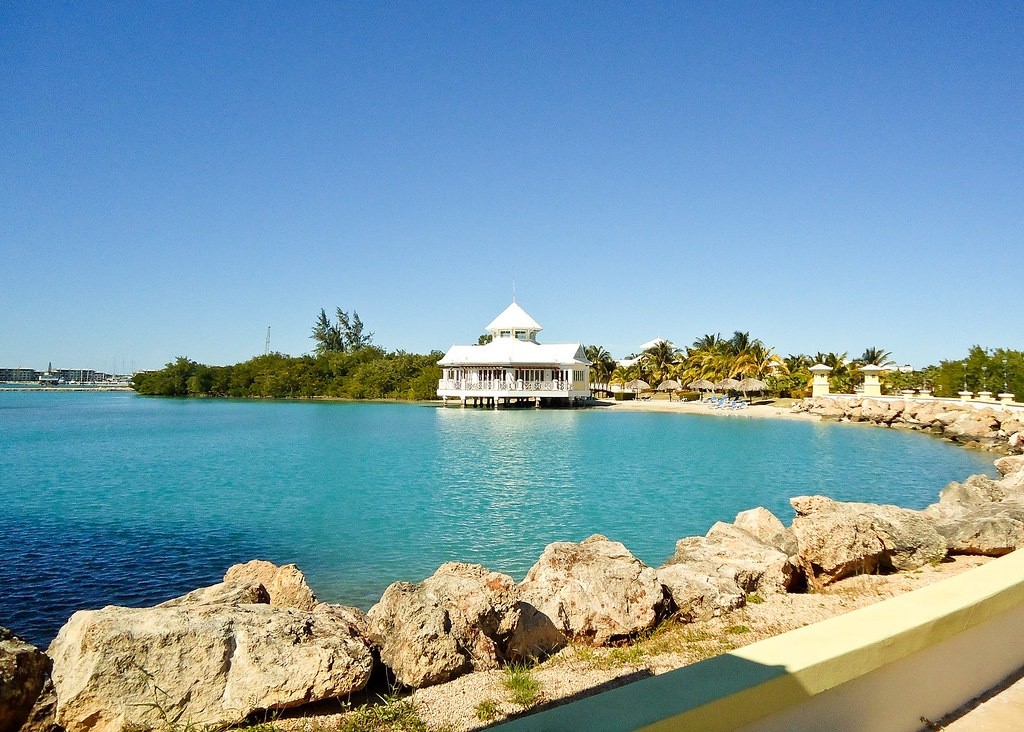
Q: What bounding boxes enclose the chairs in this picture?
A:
[708,395,746,410]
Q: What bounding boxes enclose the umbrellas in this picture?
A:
[736,377,771,405]
[715,378,741,402]
[626,379,650,401]
[657,380,682,402]
[687,379,715,402]
